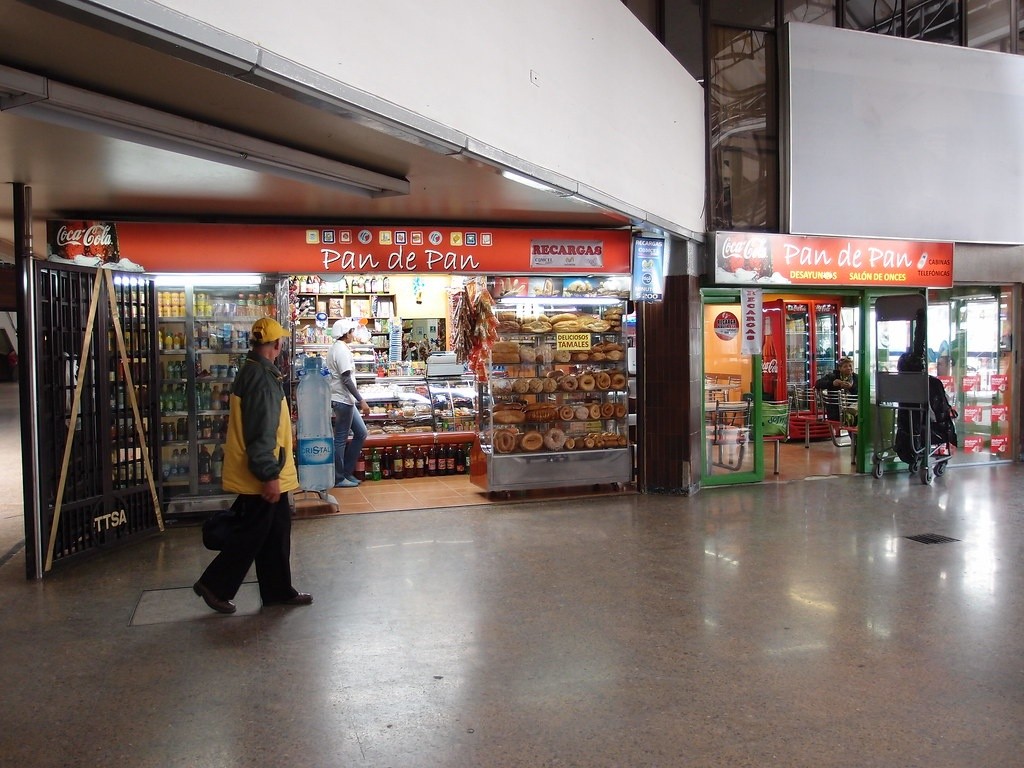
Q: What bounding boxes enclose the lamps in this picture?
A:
[0,77,410,201]
[0,62,49,110]
[114,273,279,286]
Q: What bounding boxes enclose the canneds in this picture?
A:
[157,291,212,317]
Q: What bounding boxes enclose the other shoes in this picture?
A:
[334,474,362,487]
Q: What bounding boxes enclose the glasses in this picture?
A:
[837,358,851,372]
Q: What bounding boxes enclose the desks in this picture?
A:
[704,384,739,402]
[704,401,755,471]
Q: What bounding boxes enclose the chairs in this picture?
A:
[704,376,859,475]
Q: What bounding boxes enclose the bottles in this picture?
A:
[320,369,328,376]
[761,317,778,405]
[167,361,187,379]
[817,365,833,380]
[380,403,396,410]
[817,318,824,332]
[816,337,831,358]
[787,366,805,382]
[302,352,308,358]
[307,353,309,357]
[159,328,187,349]
[312,352,317,358]
[103,323,150,488]
[299,353,305,361]
[787,345,805,358]
[160,383,230,411]
[787,318,805,331]
[352,277,389,293]
[316,354,322,368]
[435,419,475,432]
[296,357,335,491]
[163,443,225,495]
[237,293,276,315]
[162,415,229,441]
[352,443,472,482]
[295,353,306,379]
[293,276,319,294]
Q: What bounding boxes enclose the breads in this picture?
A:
[357,403,432,435]
[481,308,627,453]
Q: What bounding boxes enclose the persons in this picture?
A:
[193,317,312,614]
[326,319,370,487]
[815,357,858,439]
[936,357,957,375]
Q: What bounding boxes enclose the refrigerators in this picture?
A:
[762,299,841,442]
[150,272,289,513]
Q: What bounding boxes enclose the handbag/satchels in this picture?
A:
[202,509,241,550]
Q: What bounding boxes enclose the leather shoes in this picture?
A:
[283,591,313,605]
[193,580,236,613]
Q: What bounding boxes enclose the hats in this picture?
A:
[897,353,924,371]
[331,317,359,339]
[251,317,290,343]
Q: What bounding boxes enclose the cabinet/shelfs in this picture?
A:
[330,377,433,447]
[469,296,634,499]
[424,375,476,444]
[294,292,397,378]
[105,272,291,512]
